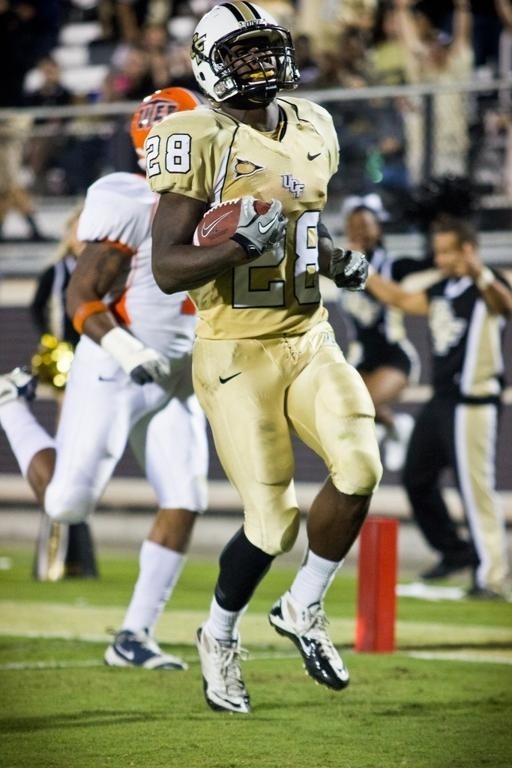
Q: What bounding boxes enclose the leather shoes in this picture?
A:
[422,545,478,580]
[469,586,497,601]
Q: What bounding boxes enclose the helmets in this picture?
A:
[130,86,208,172]
[189,1,300,104]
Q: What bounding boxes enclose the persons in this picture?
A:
[142,0,382,716]
[2,1,510,613]
[41,79,214,672]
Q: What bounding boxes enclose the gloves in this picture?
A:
[123,347,171,384]
[230,194,289,261]
[330,246,369,293]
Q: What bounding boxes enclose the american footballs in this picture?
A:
[193,199,284,250]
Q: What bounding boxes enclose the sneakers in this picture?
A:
[0,365,39,403]
[268,588,350,690]
[197,623,251,715]
[104,627,189,671]
